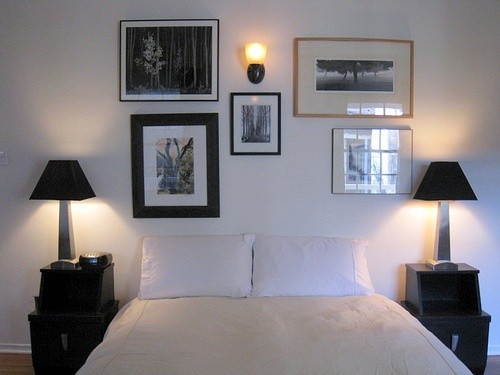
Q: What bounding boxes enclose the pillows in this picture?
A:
[139,234,254,300]
[251,235,374,296]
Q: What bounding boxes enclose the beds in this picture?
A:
[77,232,474,375]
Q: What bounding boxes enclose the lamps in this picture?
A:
[29,160,96,269]
[413,162,478,271]
[245,43,266,84]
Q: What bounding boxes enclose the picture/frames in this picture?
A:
[293,37,414,118]
[119,19,219,102]
[231,92,281,155]
[130,113,220,219]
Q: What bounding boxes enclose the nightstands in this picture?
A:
[401,262,492,375]
[28,263,119,375]
